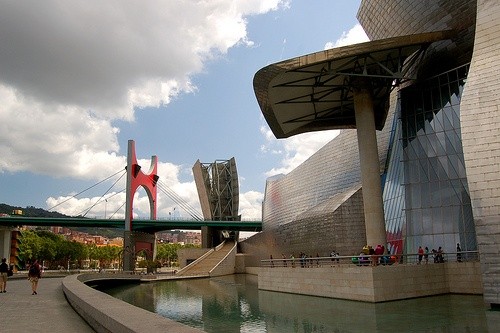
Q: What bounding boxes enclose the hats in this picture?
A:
[2,258,6,262]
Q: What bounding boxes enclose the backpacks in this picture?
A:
[31,264,37,274]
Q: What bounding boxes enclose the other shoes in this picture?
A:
[32,291,37,295]
[4,291,6,292]
[0,291,2,293]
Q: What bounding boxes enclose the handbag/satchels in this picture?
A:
[7,270,13,276]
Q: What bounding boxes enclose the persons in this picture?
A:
[431,246,447,264]
[315,253,321,268]
[281,253,288,268]
[289,253,296,268]
[424,244,430,265]
[298,252,314,268]
[27,257,41,295]
[417,245,425,266]
[0,257,8,293]
[270,255,274,267]
[455,242,463,264]
[330,250,342,267]
